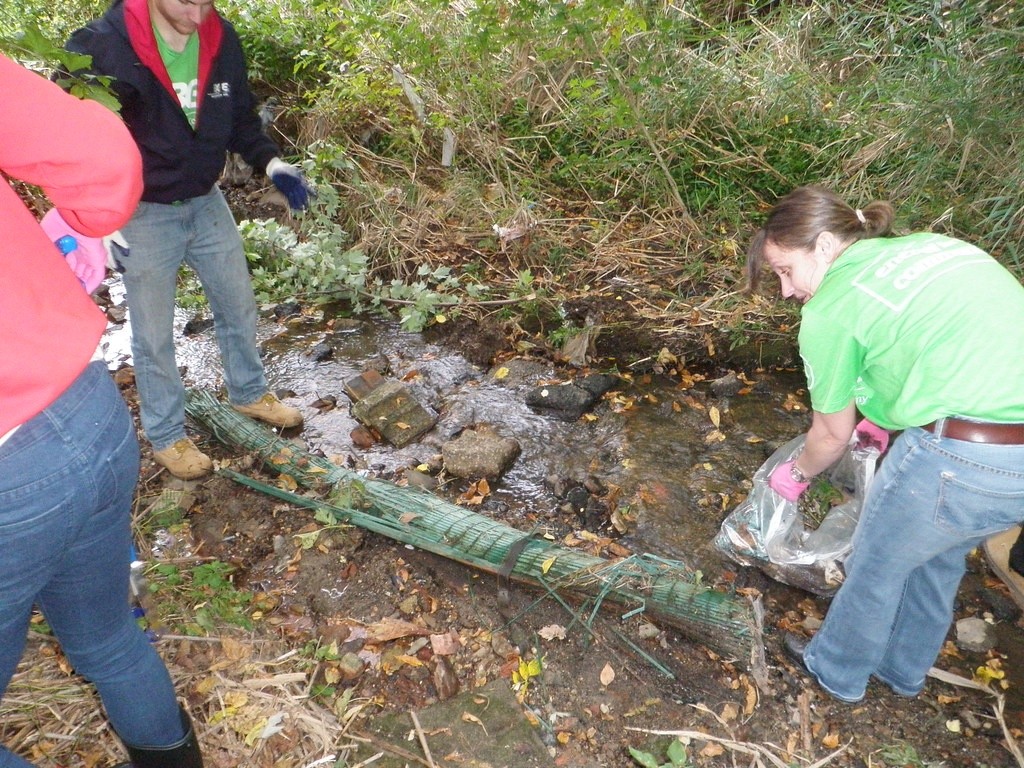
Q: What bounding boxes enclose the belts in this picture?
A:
[920,417,1024,445]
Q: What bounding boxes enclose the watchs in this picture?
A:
[791,462,818,482]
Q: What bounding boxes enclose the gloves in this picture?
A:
[855,419,889,454]
[102,229,131,274]
[40,206,108,294]
[769,460,811,502]
[265,156,318,210]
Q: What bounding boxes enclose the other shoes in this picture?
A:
[983,524,1024,611]
[781,630,818,685]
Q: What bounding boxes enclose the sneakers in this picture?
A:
[153,436,212,480]
[229,391,303,428]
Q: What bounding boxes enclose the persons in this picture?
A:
[742,189,1024,705]
[50,1,318,479]
[0,52,205,768]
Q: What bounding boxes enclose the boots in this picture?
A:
[124,710,204,768]
